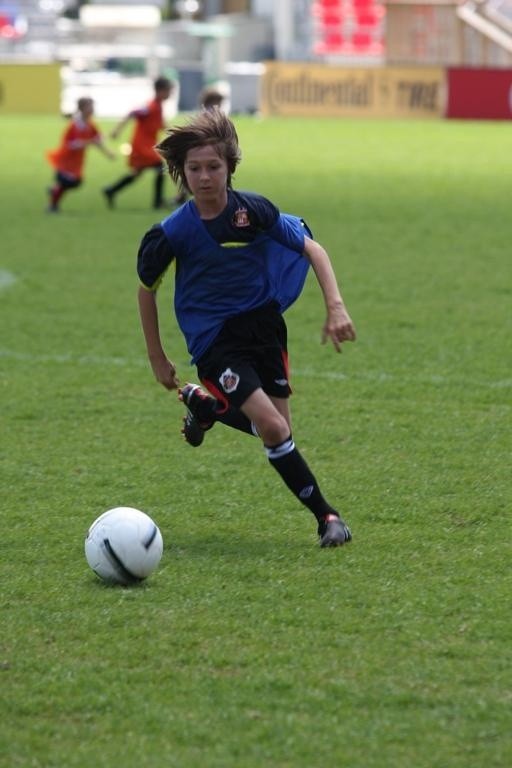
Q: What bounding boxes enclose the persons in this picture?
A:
[136,107,360,550]
[44,96,118,211]
[101,76,177,210]
[201,89,223,110]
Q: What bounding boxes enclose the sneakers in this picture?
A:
[319,510,352,546]
[177,382,215,447]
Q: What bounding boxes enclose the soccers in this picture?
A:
[84,505,163,586]
